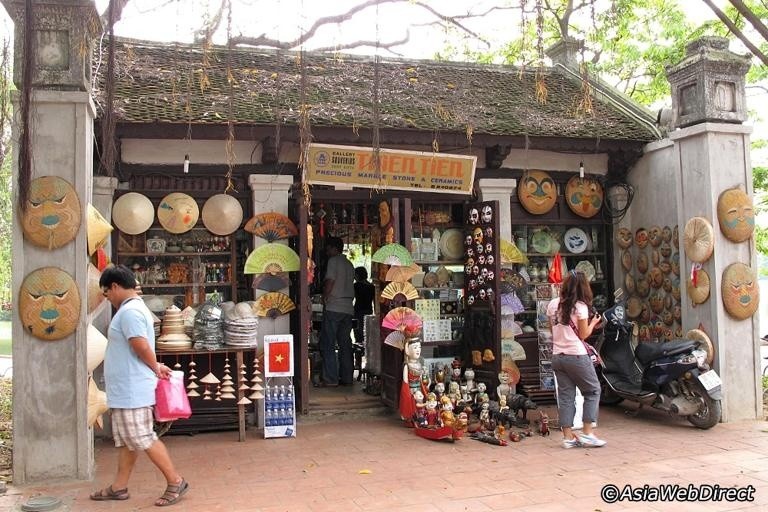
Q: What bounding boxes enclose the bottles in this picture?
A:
[265,384,293,427]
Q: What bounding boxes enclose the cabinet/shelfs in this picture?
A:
[116,172,615,441]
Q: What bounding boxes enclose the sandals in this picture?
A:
[156,477,188,506]
[90,485,128,500]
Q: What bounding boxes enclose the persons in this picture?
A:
[352,266,375,369]
[545,269,605,447]
[413,360,550,447]
[397,336,431,429]
[314,233,354,386]
[90,264,187,507]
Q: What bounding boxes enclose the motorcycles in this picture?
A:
[593,287,725,431]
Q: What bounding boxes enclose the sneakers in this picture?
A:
[563,432,606,448]
[314,380,353,387]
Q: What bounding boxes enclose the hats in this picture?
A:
[86,203,113,427]
[223,301,257,320]
[113,192,243,236]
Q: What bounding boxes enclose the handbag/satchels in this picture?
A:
[587,345,606,369]
[154,371,192,422]
